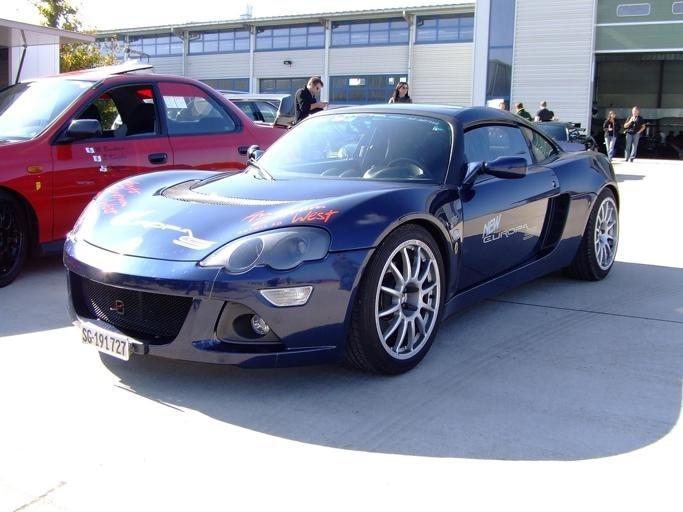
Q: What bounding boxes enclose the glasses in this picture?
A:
[399,87,408,89]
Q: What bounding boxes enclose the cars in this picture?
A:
[110,90,372,156]
[0,62,295,290]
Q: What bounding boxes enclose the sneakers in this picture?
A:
[624,158,635,163]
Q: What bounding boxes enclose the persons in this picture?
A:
[602,111,620,165]
[498,103,507,111]
[623,106,646,162]
[386,81,411,104]
[294,76,328,126]
[534,101,554,124]
[514,103,532,123]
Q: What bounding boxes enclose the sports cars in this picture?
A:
[61,102,621,377]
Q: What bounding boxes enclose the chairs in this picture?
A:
[126,102,156,136]
